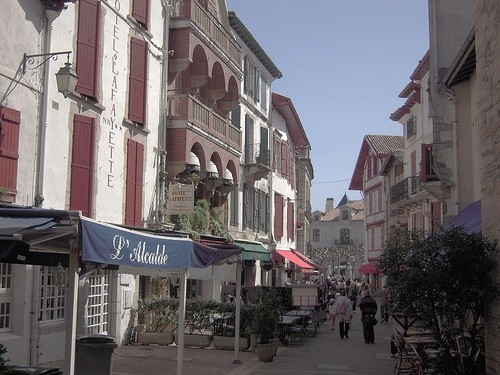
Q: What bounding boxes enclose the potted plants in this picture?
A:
[249,306,278,363]
[175,296,212,349]
[138,297,180,346]
[213,304,248,350]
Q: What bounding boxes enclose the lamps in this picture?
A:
[287,197,305,216]
[23,51,79,99]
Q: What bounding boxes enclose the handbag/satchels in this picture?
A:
[363,315,377,327]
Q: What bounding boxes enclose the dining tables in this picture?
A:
[277,316,302,341]
[286,310,314,329]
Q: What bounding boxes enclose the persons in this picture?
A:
[336,290,353,339]
[359,289,378,344]
[329,299,338,331]
[379,284,389,324]
[312,274,371,314]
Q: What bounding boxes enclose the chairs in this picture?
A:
[288,310,321,347]
[392,327,441,375]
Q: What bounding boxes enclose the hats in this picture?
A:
[330,298,336,303]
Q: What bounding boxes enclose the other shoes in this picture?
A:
[381,318,385,323]
[384,321,388,324]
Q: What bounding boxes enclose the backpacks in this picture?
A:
[338,297,348,314]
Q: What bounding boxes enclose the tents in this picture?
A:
[0,202,244,375]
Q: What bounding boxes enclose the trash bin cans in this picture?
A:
[0,363,63,375]
[75,335,119,375]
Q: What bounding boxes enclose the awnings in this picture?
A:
[291,248,323,269]
[359,264,380,273]
[438,200,481,234]
[272,249,314,269]
[214,239,271,265]
